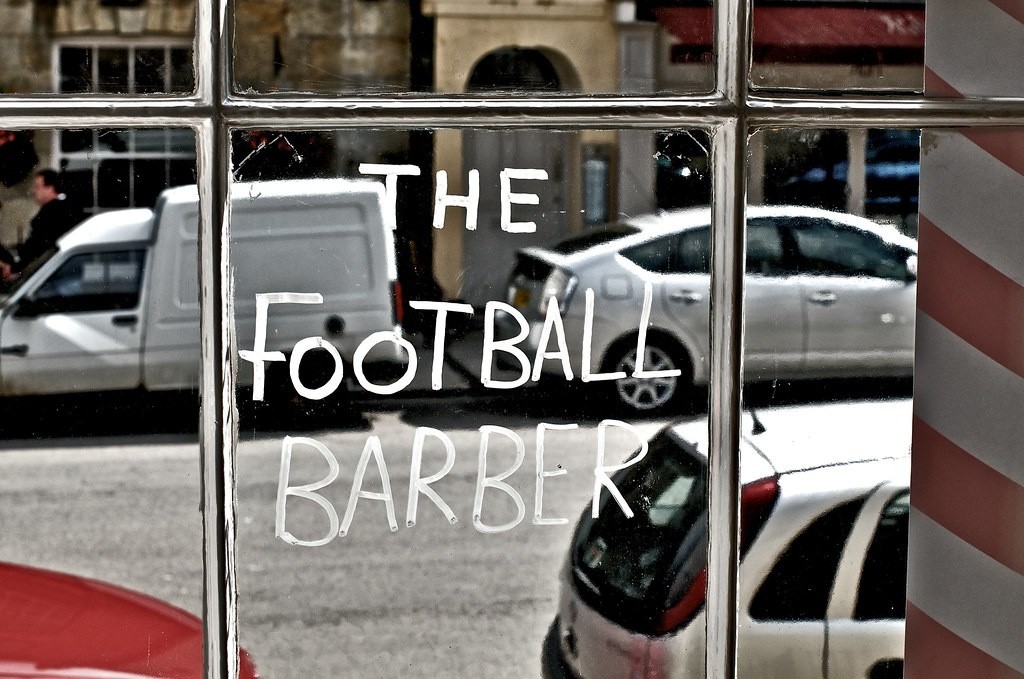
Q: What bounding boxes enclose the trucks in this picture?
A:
[0,178,407,418]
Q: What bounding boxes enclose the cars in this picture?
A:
[541,395,910,679]
[495,205,918,418]
[2,558,258,679]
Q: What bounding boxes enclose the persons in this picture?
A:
[0,169,91,295]
